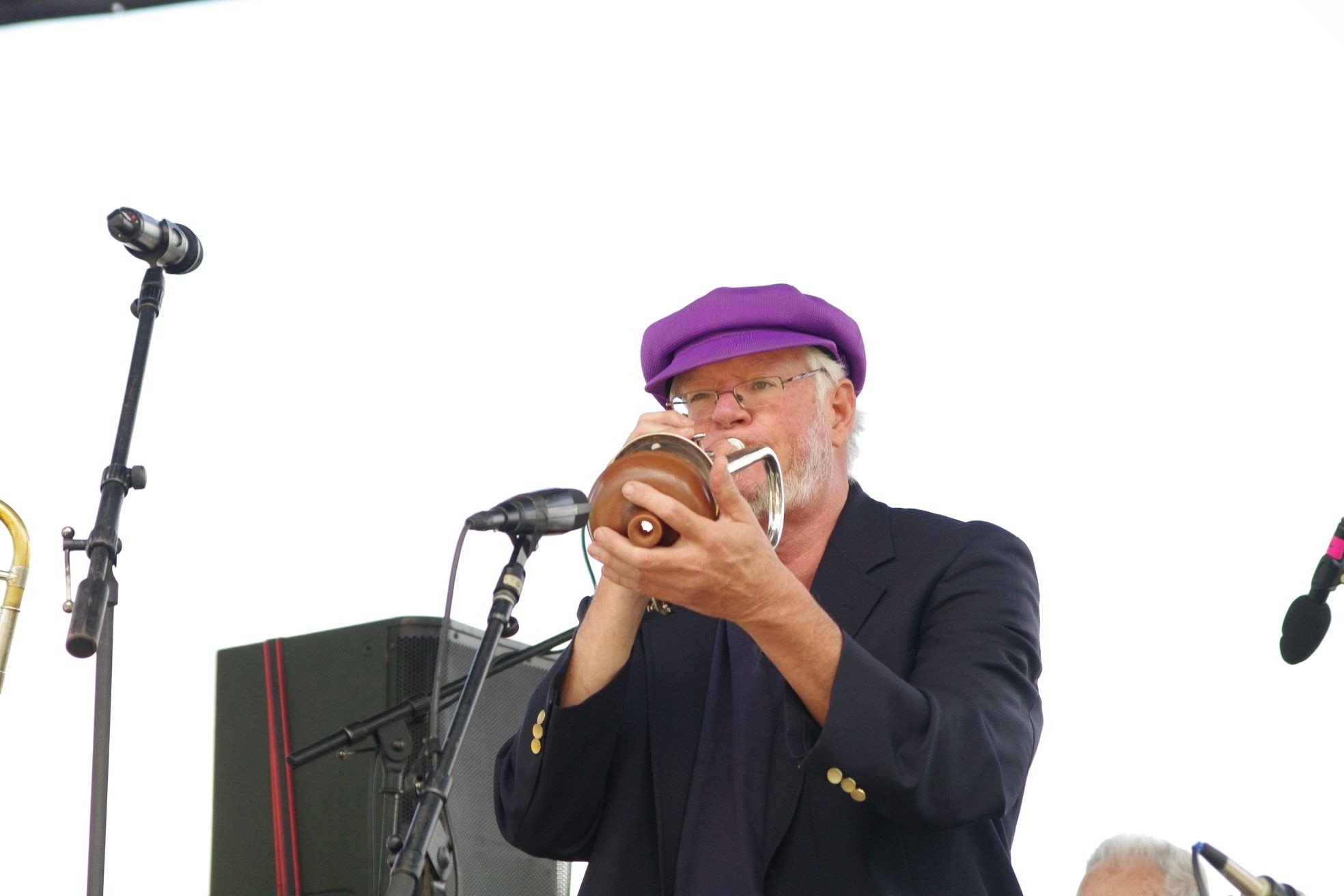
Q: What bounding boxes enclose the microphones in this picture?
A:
[1193,842,1305,896]
[467,488,591,534]
[106,208,204,275]
[1280,517,1344,665]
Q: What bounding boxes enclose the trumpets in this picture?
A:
[584,431,785,566]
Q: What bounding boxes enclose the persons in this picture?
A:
[1076,833,1207,896]
[489,283,1044,896]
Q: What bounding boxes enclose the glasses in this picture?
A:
[666,368,826,422]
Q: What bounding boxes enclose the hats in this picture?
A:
[639,282,867,413]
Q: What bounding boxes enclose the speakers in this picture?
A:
[208,615,573,896]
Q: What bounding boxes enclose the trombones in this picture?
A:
[0,499,33,696]
